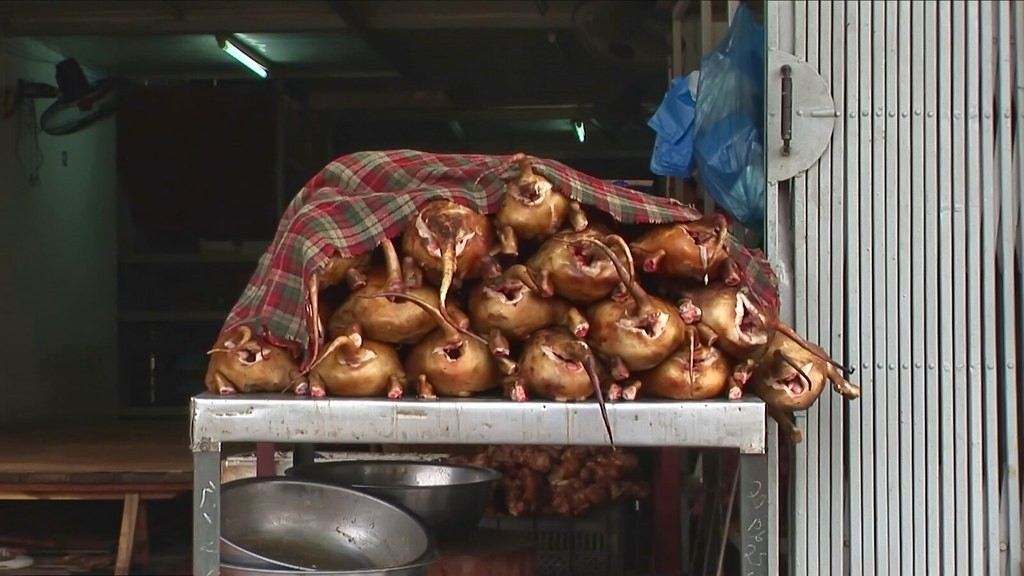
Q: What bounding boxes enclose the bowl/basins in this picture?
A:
[219,541,440,576]
[219,475,429,571]
[284,461,502,541]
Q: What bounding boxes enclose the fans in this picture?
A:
[18,57,140,138]
[571,0,673,66]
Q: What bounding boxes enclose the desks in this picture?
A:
[190,385,769,576]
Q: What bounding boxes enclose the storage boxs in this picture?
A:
[477,474,647,576]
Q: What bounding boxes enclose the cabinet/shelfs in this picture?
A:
[119,83,337,412]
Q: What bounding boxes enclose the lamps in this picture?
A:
[214,27,293,81]
[448,116,468,146]
[574,118,586,143]
